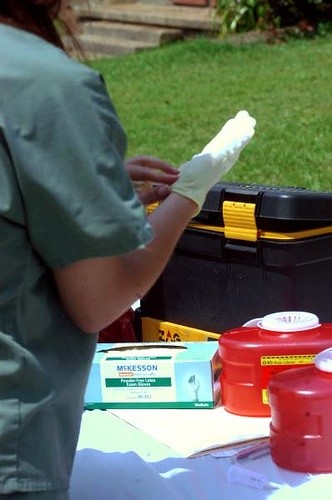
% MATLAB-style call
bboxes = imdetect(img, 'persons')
[0,0,256,500]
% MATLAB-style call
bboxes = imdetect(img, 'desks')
[67,402,332,500]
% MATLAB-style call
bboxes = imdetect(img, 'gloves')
[161,110,257,219]
